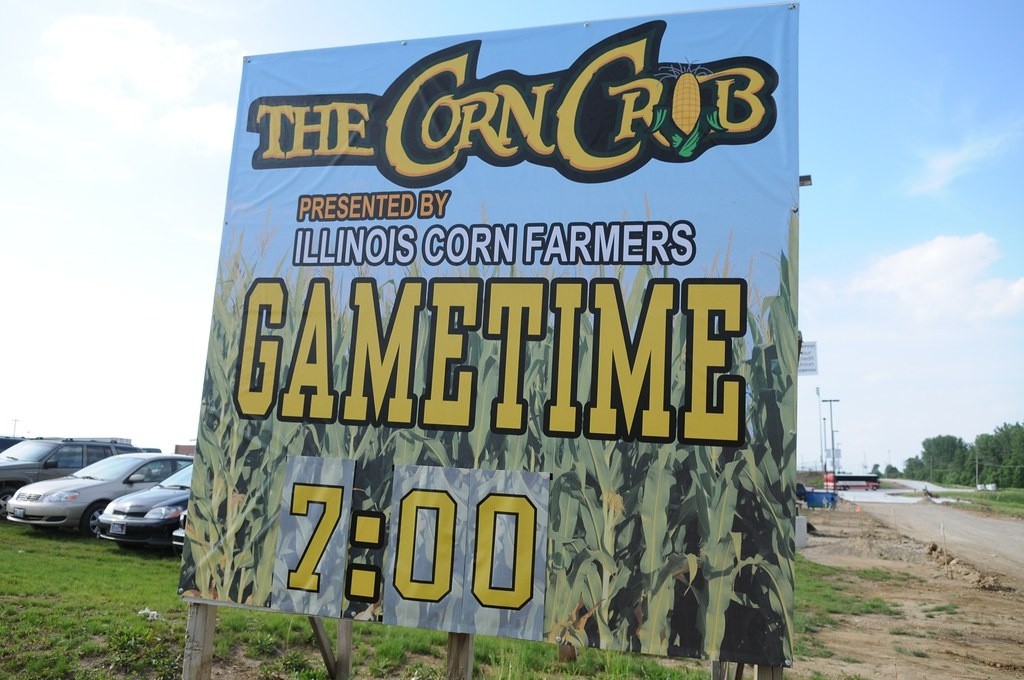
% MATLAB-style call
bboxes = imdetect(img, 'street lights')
[822,400,839,492]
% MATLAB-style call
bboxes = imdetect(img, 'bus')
[823,474,880,491]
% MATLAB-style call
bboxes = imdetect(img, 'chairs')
[142,467,151,482]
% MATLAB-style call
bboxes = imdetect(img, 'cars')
[0,437,153,518]
[7,452,195,537]
[97,463,194,548]
[172,510,187,556]
